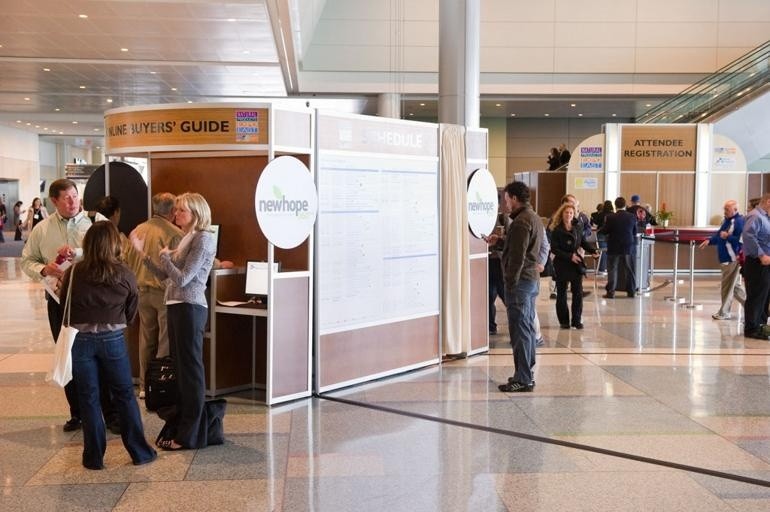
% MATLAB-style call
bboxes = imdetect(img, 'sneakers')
[712,313,730,319]
[498,377,534,392]
[63,417,81,432]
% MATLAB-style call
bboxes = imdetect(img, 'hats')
[631,195,639,202]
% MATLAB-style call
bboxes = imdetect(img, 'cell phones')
[481,234,491,242]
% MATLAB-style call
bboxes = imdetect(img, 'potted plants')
[656,202,673,227]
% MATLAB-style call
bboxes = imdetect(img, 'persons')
[699,193,769,340]
[1,198,50,243]
[547,147,559,170]
[19,180,219,469]
[557,143,570,167]
[481,181,658,393]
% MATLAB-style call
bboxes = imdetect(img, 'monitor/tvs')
[244,259,282,304]
[211,224,221,258]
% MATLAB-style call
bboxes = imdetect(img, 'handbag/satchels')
[204,399,227,445]
[145,355,178,410]
[45,325,80,389]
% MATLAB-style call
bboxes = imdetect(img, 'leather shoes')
[159,440,183,450]
